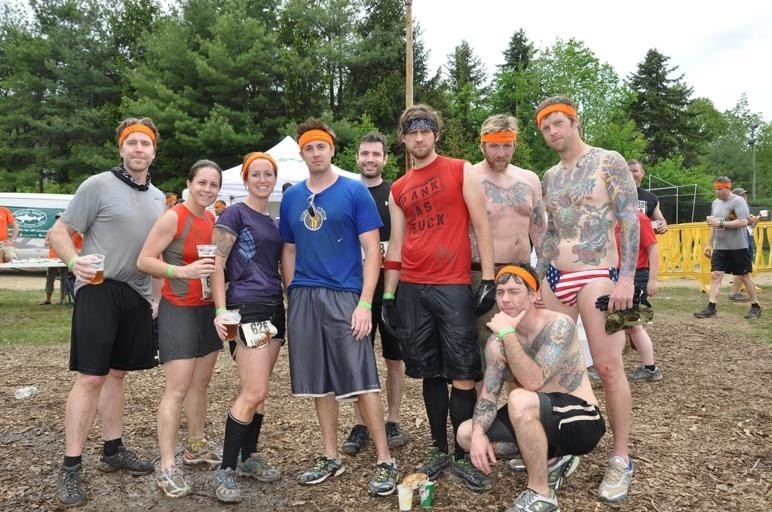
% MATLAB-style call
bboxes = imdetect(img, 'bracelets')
[11,240,16,242]
[719,220,724,229]
[499,327,517,341]
[358,301,372,311]
[383,293,394,299]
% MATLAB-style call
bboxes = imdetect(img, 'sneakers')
[626,367,665,382]
[296,455,347,487]
[727,292,748,301]
[542,454,581,492]
[95,447,153,477]
[505,487,562,512]
[693,308,718,318]
[180,440,225,466]
[746,306,762,319]
[340,424,372,456]
[450,452,495,493]
[234,451,283,484]
[55,463,88,507]
[598,451,634,503]
[153,460,193,499]
[366,456,401,496]
[211,463,244,504]
[383,421,408,450]
[417,445,451,480]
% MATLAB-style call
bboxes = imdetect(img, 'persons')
[535,97,667,499]
[44,118,166,507]
[729,187,768,302]
[464,113,549,392]
[383,104,496,490]
[693,176,762,319]
[457,264,606,512]
[137,159,224,499]
[0,206,19,263]
[343,133,405,456]
[211,152,284,502]
[280,120,399,494]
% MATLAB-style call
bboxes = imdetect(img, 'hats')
[733,186,751,195]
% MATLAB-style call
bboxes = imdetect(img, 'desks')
[1,259,68,305]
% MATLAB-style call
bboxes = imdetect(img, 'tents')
[183,136,359,215]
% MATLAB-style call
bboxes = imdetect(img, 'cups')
[706,216,713,226]
[397,485,413,510]
[420,480,434,507]
[219,310,241,341]
[652,220,663,234]
[196,244,217,274]
[83,254,105,285]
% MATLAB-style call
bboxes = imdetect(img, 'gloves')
[472,278,498,316]
[379,297,400,331]
[593,291,645,311]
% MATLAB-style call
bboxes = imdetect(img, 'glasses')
[306,192,318,217]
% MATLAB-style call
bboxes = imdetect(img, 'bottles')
[14,386,38,400]
